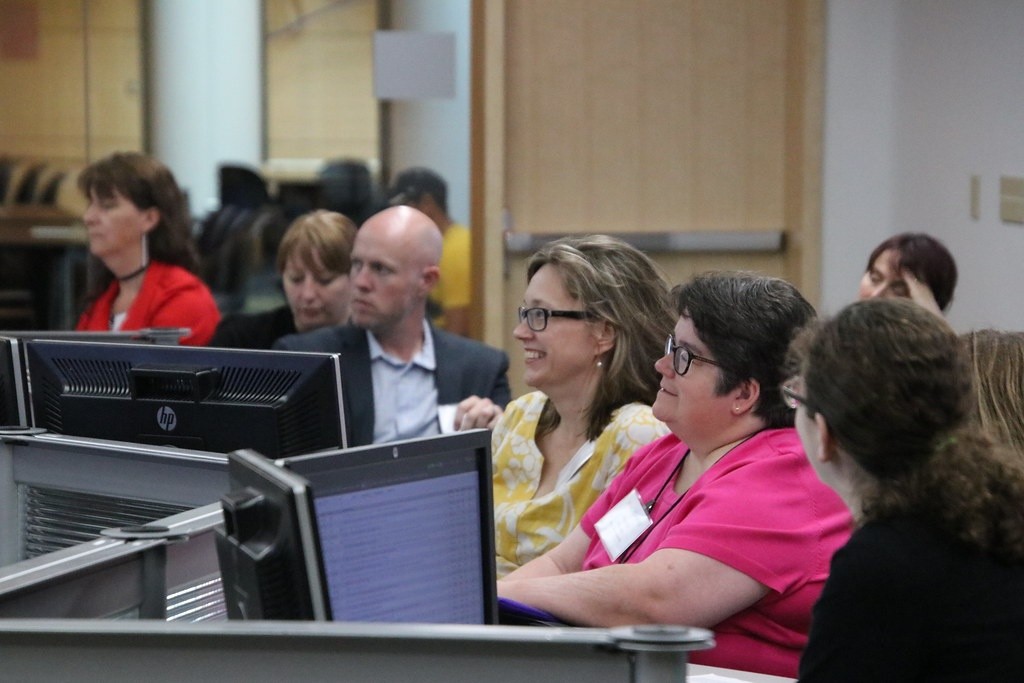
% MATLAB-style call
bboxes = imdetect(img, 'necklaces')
[116,259,152,282]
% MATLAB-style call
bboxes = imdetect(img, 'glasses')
[780,377,831,434]
[518,306,594,332]
[664,333,747,376]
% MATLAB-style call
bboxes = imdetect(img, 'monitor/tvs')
[0,335,34,430]
[213,448,332,622]
[25,338,351,459]
[273,426,498,625]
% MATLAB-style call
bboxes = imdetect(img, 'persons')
[63,147,1024,683]
